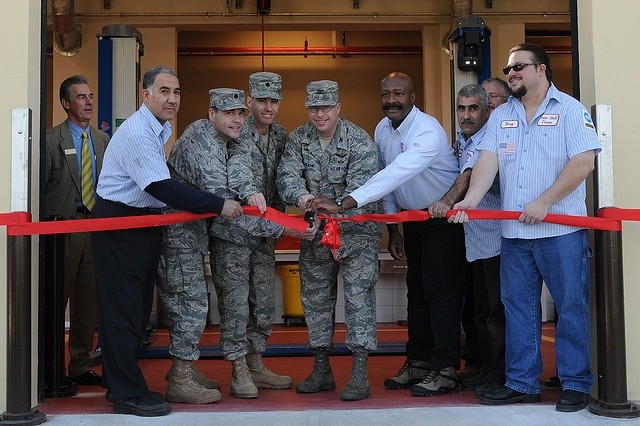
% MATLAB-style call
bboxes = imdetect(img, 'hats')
[249,72,283,99]
[305,80,339,107]
[209,88,249,111]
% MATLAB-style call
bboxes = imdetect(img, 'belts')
[97,196,164,214]
[77,206,92,214]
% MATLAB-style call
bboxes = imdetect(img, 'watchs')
[334,197,345,214]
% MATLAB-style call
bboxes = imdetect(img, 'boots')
[166,358,221,404]
[230,356,258,398]
[342,354,370,400]
[296,352,336,392]
[246,353,292,389]
[193,361,220,389]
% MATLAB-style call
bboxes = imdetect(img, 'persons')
[207,69,295,400]
[479,76,511,118]
[155,86,318,406]
[44,73,111,399]
[427,83,505,396]
[276,77,381,403]
[304,69,464,398]
[89,64,245,418]
[446,43,604,416]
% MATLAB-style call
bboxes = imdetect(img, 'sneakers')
[384,359,431,389]
[411,366,462,396]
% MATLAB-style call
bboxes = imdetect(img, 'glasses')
[306,103,339,113]
[502,63,541,75]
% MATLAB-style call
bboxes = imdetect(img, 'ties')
[82,132,95,210]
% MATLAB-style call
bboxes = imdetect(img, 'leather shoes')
[113,394,172,416]
[462,371,490,391]
[475,379,506,398]
[461,365,476,378]
[79,370,103,384]
[106,390,164,402]
[481,386,541,405]
[556,389,591,412]
